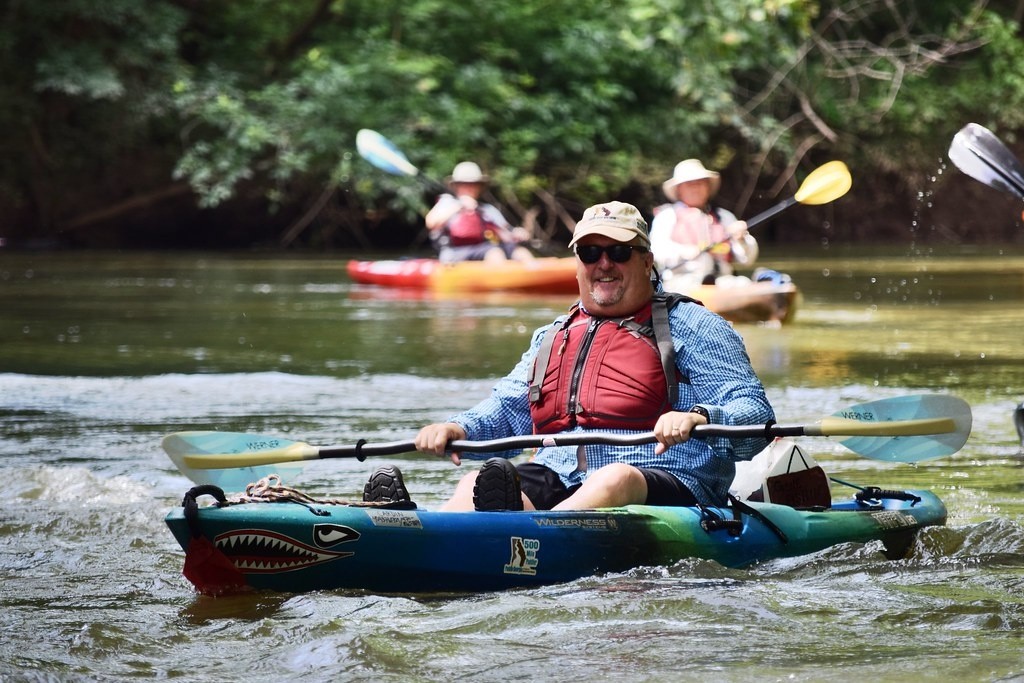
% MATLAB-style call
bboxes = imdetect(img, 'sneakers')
[363,466,410,504]
[473,456,523,511]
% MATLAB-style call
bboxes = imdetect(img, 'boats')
[665,280,798,324]
[348,255,579,294]
[164,483,947,596]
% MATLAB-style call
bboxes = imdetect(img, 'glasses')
[572,244,649,264]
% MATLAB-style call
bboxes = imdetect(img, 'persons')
[363,200,777,511]
[426,161,535,260]
[649,158,759,285]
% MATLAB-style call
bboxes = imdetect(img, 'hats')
[662,158,720,201]
[445,162,490,188]
[567,201,651,250]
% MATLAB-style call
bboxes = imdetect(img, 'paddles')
[948,121,1024,199]
[658,157,853,280]
[162,393,972,495]
[356,129,553,253]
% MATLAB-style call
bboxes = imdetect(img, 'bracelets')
[690,406,710,424]
